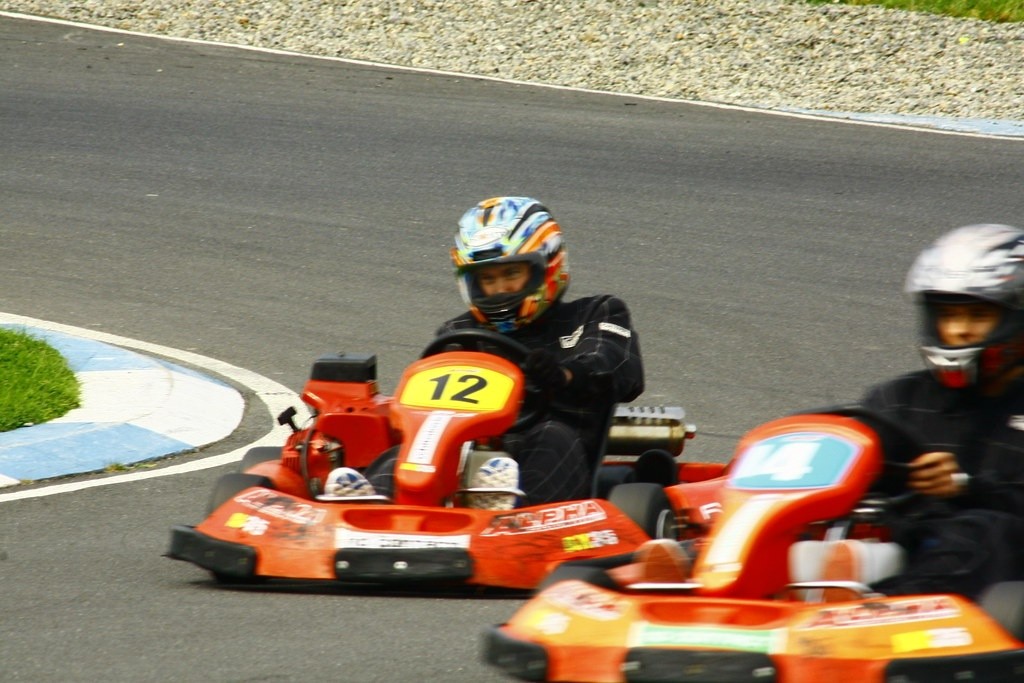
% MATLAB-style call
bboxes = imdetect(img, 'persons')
[324,195,645,511]
[639,224,1024,600]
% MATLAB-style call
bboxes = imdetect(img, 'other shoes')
[820,539,859,602]
[465,456,523,510]
[641,538,687,583]
[325,468,375,497]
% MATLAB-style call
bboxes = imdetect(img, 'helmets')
[903,223,1024,386]
[450,197,571,333]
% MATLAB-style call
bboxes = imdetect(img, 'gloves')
[522,348,567,390]
